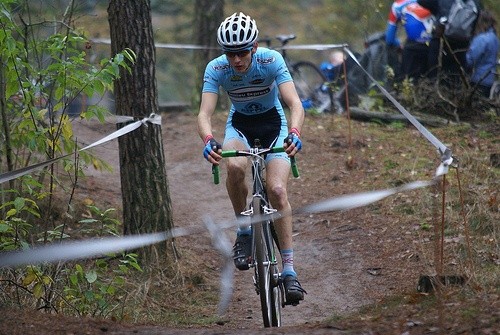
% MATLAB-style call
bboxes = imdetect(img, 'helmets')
[216,11,259,51]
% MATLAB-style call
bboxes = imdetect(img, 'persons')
[196,11,308,301]
[313,51,347,115]
[384,0,500,98]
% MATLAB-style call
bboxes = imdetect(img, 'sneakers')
[231,227,252,270]
[284,275,307,301]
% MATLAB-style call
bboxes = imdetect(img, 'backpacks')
[445,0,478,38]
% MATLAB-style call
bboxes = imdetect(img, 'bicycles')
[257,34,334,116]
[211,147,299,329]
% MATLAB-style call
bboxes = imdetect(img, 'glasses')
[223,46,253,58]
